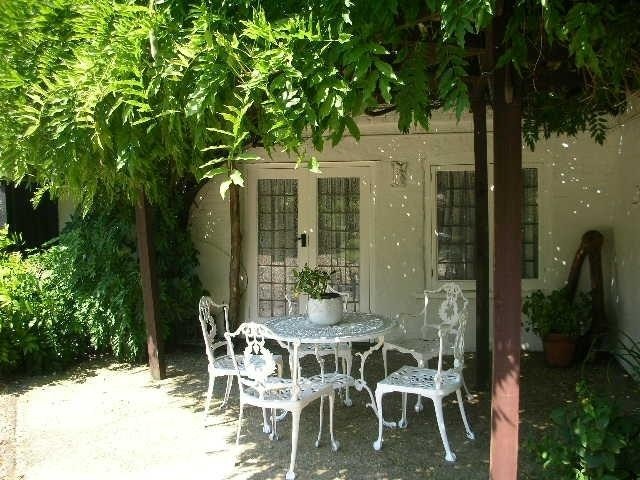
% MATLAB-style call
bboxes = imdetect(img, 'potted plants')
[290,264,347,326]
[520,286,596,367]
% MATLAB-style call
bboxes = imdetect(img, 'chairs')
[369,309,477,463]
[196,297,287,438]
[380,282,477,415]
[284,283,357,406]
[224,320,340,479]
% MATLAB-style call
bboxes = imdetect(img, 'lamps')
[390,159,408,187]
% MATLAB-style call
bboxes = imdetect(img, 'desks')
[250,310,403,451]
